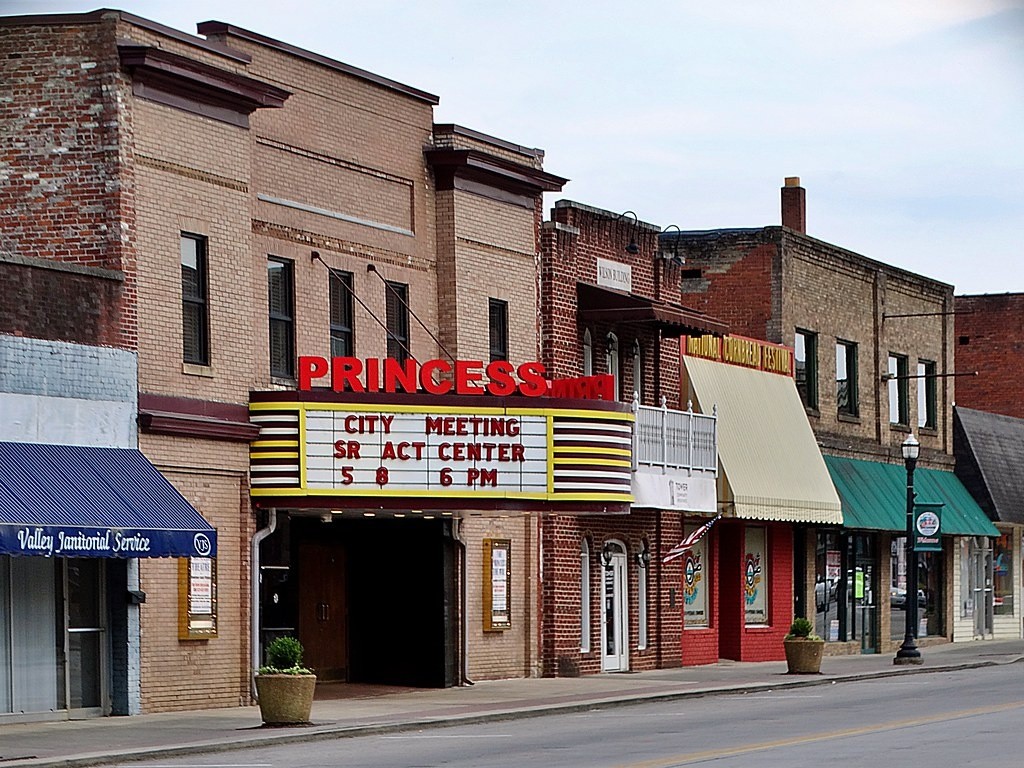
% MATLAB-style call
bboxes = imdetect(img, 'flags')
[664,511,722,562]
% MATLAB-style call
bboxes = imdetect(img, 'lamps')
[638,225,685,267]
[592,211,639,255]
[596,543,615,569]
[634,545,652,569]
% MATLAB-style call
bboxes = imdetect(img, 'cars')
[814,573,928,613]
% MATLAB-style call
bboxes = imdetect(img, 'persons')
[0,441,217,558]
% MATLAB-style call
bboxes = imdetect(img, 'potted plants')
[783,618,824,674]
[253,638,317,724]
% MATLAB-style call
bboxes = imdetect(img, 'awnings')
[953,407,1024,526]
[822,454,1001,540]
[681,357,843,523]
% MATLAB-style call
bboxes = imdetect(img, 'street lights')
[889,432,926,664]
